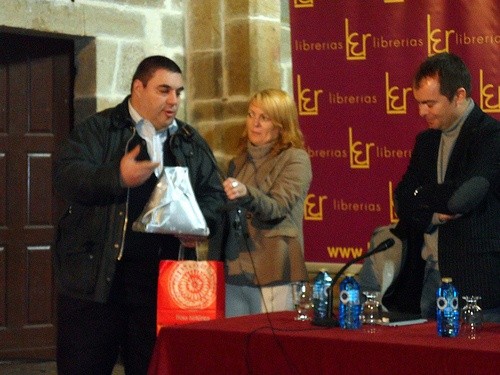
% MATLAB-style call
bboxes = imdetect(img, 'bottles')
[312,269,334,326]
[339,273,361,328]
[435,278,459,336]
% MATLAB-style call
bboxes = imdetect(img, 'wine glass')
[292,281,312,322]
[460,295,484,339]
[358,291,384,332]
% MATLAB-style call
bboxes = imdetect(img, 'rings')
[232,181,238,187]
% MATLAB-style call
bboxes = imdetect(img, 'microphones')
[320,237,396,329]
[227,161,248,238]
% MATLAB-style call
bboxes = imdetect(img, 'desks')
[143,308,500,375]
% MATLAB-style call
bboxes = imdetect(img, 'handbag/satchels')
[225,198,248,260]
[157,235,226,337]
[360,222,404,290]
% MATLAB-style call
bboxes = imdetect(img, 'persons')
[220,88,313,319]
[51,56,226,375]
[382,52,500,313]
